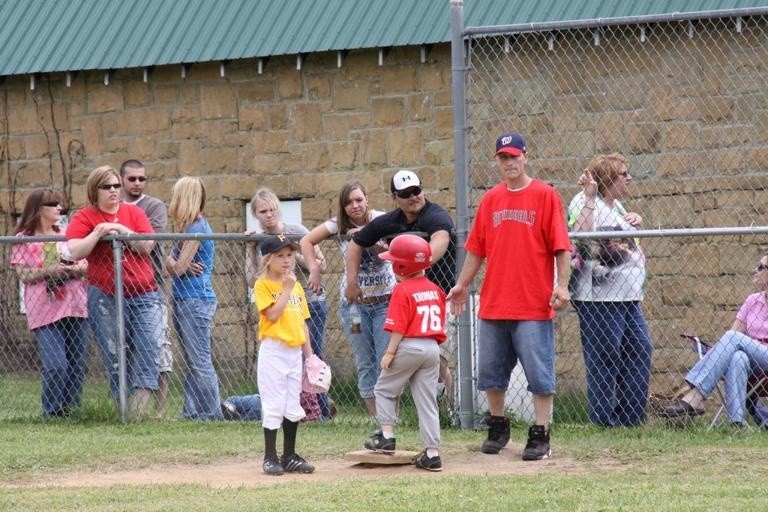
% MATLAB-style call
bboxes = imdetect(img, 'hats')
[391,171,421,193]
[261,235,291,255]
[494,134,526,159]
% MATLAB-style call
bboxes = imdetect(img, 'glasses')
[98,184,121,189]
[43,201,60,206]
[395,189,420,197]
[128,176,145,181]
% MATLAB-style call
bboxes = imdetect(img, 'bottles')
[54,249,76,285]
[349,300,362,335]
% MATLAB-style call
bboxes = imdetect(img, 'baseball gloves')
[302,353,332,393]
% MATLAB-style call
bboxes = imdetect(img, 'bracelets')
[584,205,596,210]
[385,351,394,356]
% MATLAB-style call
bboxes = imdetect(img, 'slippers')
[661,400,704,417]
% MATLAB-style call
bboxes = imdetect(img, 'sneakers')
[263,453,314,475]
[481,415,510,454]
[523,424,551,460]
[415,453,441,470]
[365,431,395,452]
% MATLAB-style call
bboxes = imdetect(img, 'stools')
[681,329,767,432]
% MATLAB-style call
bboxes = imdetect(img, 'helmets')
[378,235,433,276]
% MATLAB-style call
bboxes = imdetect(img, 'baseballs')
[551,297,561,305]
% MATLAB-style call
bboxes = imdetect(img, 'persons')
[658,254,768,436]
[66,166,160,414]
[252,234,334,475]
[565,151,655,426]
[166,175,224,422]
[9,189,89,416]
[299,180,404,426]
[364,233,446,471]
[117,159,173,420]
[445,132,573,462]
[245,186,330,419]
[344,170,457,410]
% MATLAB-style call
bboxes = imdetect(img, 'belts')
[362,294,392,304]
